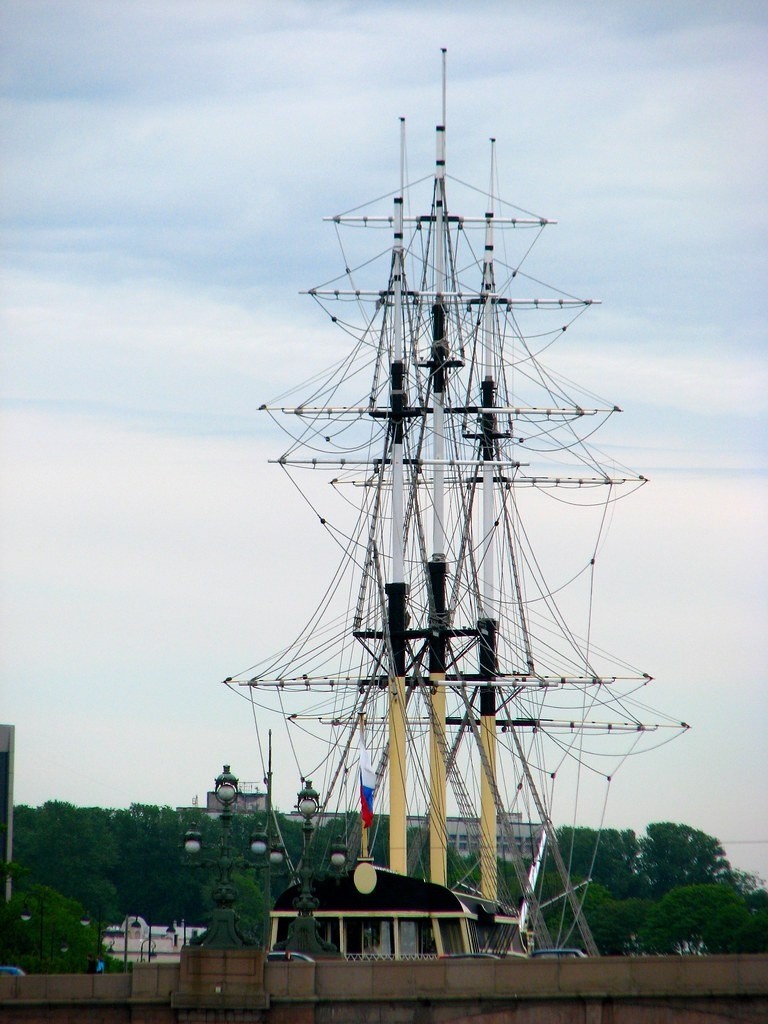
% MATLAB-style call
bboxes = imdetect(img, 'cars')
[269,952,316,962]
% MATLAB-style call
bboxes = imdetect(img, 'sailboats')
[222,48,679,962]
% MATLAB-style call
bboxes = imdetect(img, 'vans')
[439,953,502,962]
[529,950,589,961]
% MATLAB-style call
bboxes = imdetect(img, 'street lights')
[131,903,152,963]
[270,781,348,953]
[178,766,269,947]
[79,898,101,956]
[138,938,157,962]
[21,892,43,958]
[166,914,187,945]
